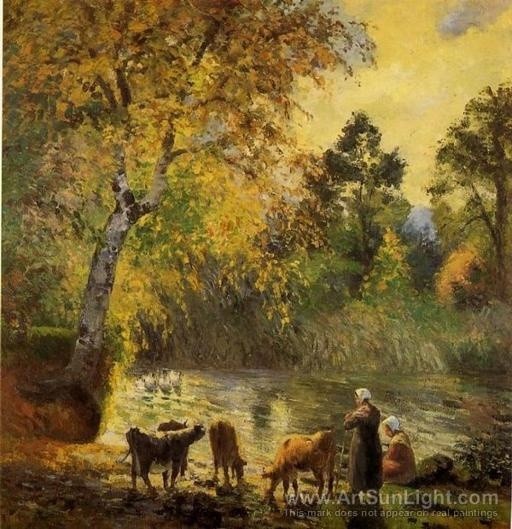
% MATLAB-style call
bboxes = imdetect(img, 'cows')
[119,417,206,490]
[259,429,337,502]
[207,419,248,484]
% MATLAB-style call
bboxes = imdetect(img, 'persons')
[342,388,384,506]
[383,415,416,485]
